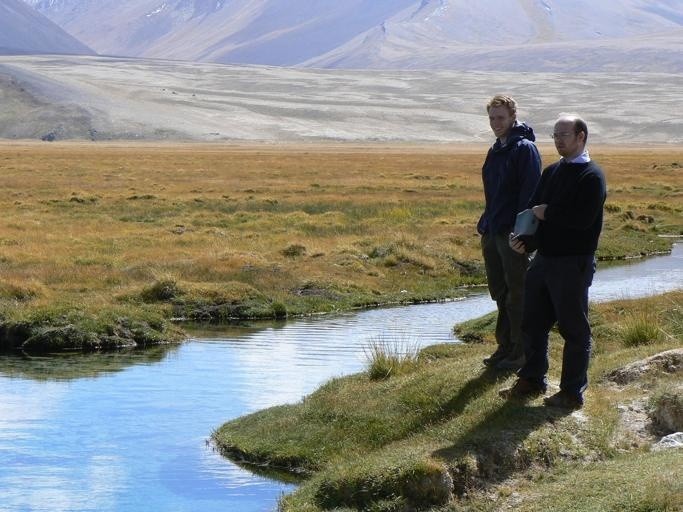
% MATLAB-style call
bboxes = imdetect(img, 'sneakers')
[499,378,546,396]
[484,350,520,370]
[544,391,583,407]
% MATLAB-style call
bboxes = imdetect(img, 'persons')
[475,95,543,370]
[495,114,608,409]
